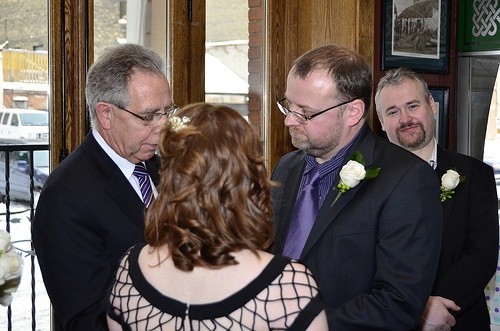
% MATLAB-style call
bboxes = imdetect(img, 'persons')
[374,69,499,331]
[32,46,180,331]
[263,46,438,331]
[106,103,330,331]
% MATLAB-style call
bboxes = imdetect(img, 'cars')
[0,138,50,208]
[0,105,48,141]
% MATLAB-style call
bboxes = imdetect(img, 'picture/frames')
[379,0,451,75]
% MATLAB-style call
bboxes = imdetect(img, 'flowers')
[0,229,22,307]
[330,151,382,208]
[440,166,466,202]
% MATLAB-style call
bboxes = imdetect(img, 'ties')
[282,166,321,261]
[134,162,156,212]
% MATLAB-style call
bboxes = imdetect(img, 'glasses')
[277,98,360,124]
[105,100,180,126]
